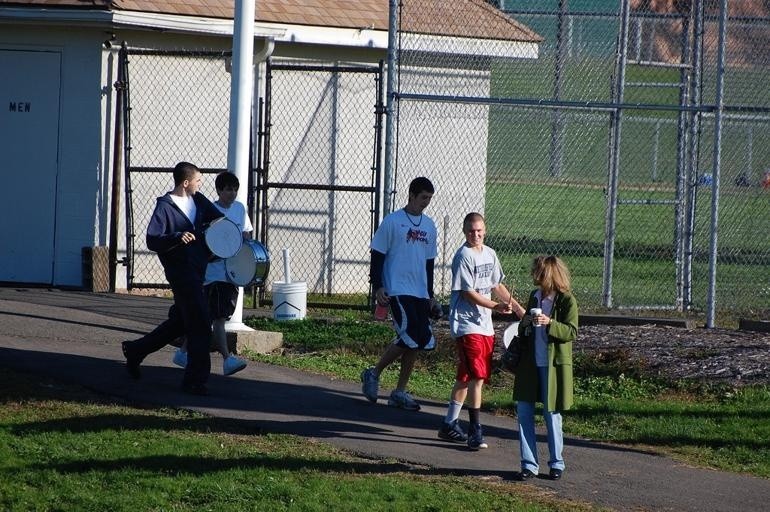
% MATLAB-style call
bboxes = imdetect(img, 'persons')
[513,254,577,483]
[172,172,254,377]
[438,212,526,451]
[121,162,225,397]
[359,176,438,410]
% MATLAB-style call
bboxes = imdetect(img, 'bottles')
[374,291,391,322]
[427,303,441,326]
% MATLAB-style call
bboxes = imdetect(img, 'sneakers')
[120,339,147,382]
[386,389,421,411]
[222,355,246,376]
[359,367,380,404]
[173,348,189,368]
[467,423,488,450]
[180,380,208,397]
[436,415,469,443]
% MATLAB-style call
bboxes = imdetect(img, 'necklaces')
[404,206,423,227]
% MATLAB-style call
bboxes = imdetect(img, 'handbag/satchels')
[501,335,525,376]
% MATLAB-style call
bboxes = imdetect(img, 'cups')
[530,307,543,327]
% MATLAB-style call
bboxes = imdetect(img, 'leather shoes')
[549,466,562,480]
[517,468,535,479]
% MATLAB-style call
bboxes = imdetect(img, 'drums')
[502,321,522,373]
[225,239,269,287]
[199,218,242,264]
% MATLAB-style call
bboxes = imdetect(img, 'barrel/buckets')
[272,281,308,321]
